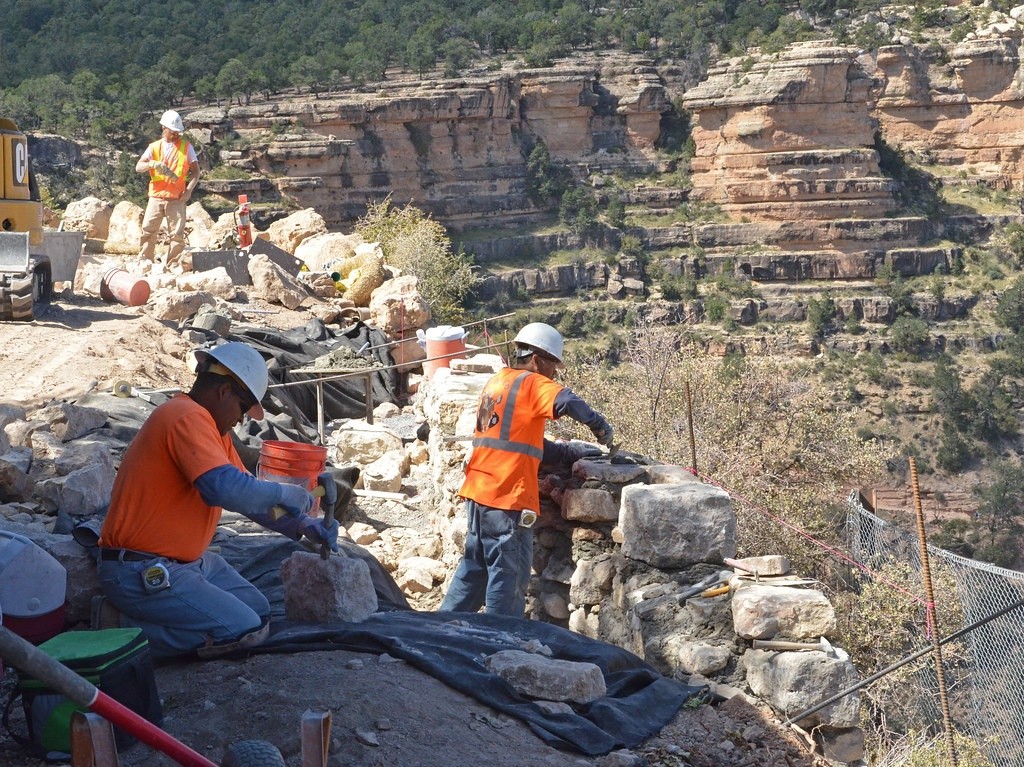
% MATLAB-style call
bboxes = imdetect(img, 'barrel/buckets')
[256,439,327,514]
[101,268,150,306]
[425,325,470,380]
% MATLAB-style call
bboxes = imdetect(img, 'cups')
[165,168,178,182]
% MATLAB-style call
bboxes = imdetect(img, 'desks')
[291,365,373,426]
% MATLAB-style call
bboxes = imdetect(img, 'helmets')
[195,342,269,420]
[511,322,565,369]
[159,110,185,132]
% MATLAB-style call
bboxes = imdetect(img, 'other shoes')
[90,595,121,632]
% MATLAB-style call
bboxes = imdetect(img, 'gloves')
[298,513,340,552]
[273,482,314,519]
[148,160,167,174]
[590,419,614,448]
[561,441,603,464]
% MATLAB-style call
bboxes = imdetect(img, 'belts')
[100,548,156,562]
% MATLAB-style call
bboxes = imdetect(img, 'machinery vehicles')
[0,117,87,322]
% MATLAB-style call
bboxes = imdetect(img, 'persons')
[135,109,200,266]
[90,341,340,660]
[438,322,614,621]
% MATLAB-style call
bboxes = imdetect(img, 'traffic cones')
[238,193,253,249]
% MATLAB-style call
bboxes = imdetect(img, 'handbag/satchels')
[2,624,164,765]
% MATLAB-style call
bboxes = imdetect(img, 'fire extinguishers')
[233,201,252,249]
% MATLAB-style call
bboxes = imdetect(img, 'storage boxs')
[17,627,165,755]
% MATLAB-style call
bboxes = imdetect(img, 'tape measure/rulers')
[140,563,171,591]
[516,509,537,528]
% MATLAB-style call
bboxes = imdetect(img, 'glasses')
[229,389,251,415]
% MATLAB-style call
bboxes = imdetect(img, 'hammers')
[722,557,760,584]
[751,635,835,657]
[267,472,338,522]
[596,428,623,460]
